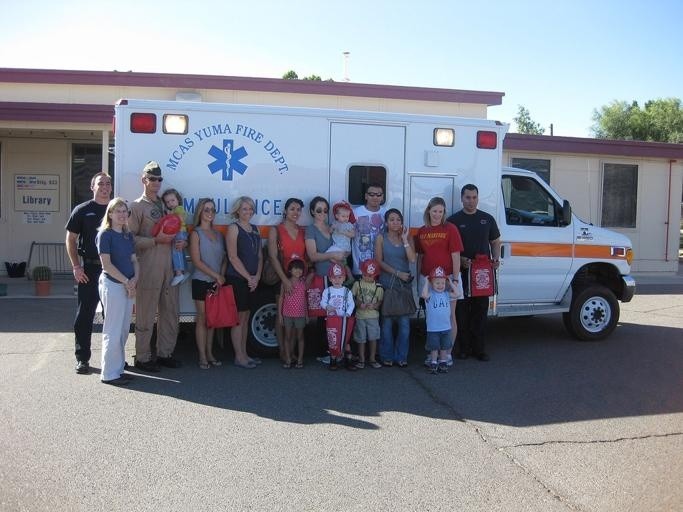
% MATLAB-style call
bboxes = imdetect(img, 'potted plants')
[32,265,52,297]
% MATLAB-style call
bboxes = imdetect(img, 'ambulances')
[111,93,638,363]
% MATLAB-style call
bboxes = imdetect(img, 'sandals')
[206,357,224,368]
[197,357,210,370]
[279,353,304,370]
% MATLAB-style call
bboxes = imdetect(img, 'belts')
[85,257,102,265]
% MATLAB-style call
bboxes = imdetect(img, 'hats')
[359,259,381,275]
[327,263,348,277]
[143,160,161,176]
[427,265,449,279]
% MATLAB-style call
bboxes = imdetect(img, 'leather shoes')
[135,359,161,373]
[233,356,263,369]
[156,353,178,370]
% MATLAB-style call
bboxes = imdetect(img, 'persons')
[65,161,500,385]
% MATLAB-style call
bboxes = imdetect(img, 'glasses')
[145,177,163,182]
[367,192,384,196]
[203,208,217,213]
[315,208,328,214]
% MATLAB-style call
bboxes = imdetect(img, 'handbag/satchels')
[260,224,285,286]
[324,307,356,358]
[381,270,417,316]
[467,253,499,299]
[305,272,328,318]
[204,282,240,329]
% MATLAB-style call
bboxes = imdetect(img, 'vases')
[4,261,26,278]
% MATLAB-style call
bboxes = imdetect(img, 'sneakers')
[179,272,191,286]
[424,346,490,375]
[327,359,408,372]
[171,273,184,287]
[75,358,90,374]
[101,372,136,386]
